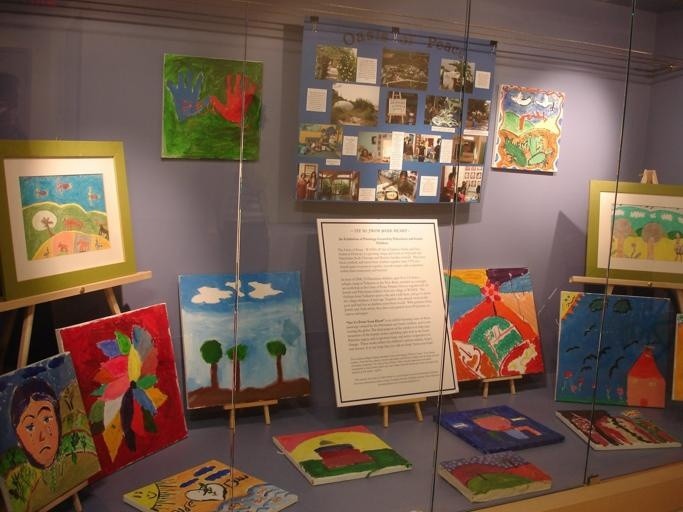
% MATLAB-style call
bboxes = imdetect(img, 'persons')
[3,378,61,512]
[297,97,491,203]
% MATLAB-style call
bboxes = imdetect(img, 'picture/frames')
[585,180,683,285]
[0,138,136,302]
